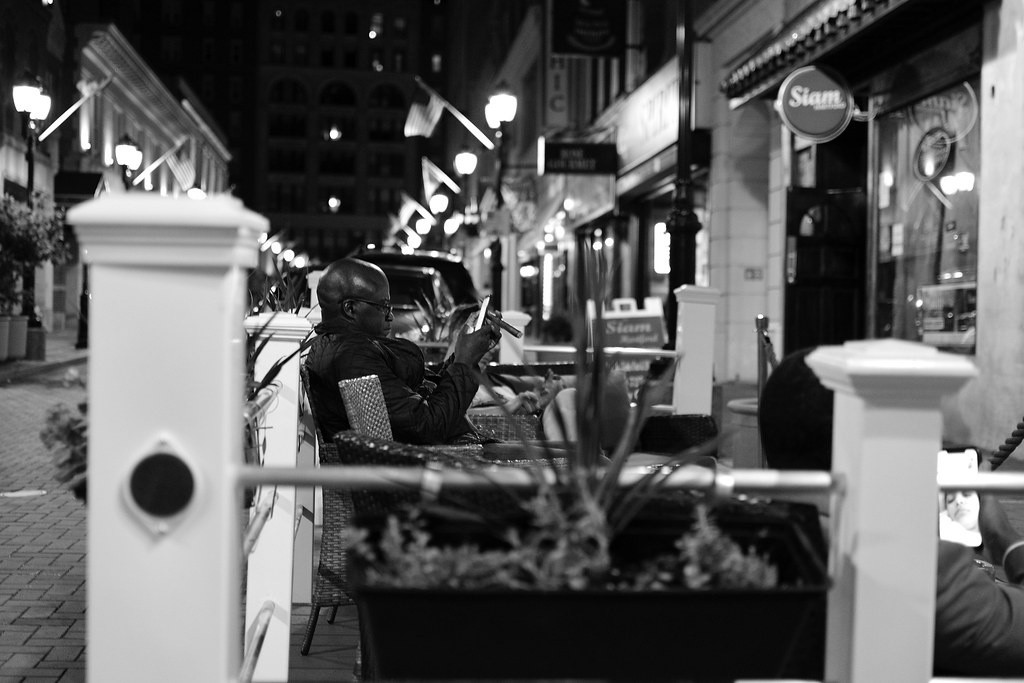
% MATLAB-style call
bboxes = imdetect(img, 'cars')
[354,246,484,340]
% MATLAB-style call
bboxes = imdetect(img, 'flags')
[382,156,446,251]
[404,76,447,137]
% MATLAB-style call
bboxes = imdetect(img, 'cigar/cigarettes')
[485,308,523,339]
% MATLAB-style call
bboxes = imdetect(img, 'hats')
[758,350,834,471]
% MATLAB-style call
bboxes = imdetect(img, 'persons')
[945,489,979,531]
[756,347,1024,683]
[304,258,613,467]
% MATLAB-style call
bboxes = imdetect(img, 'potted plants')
[343,245,831,683]
[0,191,70,362]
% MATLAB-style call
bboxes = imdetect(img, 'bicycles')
[400,285,526,356]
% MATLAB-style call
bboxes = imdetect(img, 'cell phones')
[475,294,492,332]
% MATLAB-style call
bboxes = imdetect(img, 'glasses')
[351,298,391,321]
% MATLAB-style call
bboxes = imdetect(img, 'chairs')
[298,365,565,654]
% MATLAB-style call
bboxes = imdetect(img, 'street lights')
[115,133,144,192]
[479,79,518,321]
[11,68,53,329]
